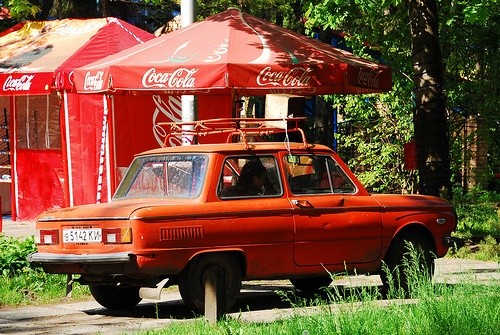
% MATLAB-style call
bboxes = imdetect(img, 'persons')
[223,160,270,197]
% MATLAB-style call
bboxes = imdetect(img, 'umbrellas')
[74,7,393,143]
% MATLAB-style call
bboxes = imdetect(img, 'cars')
[27,115,459,316]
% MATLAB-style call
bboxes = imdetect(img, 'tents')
[0,17,237,223]
